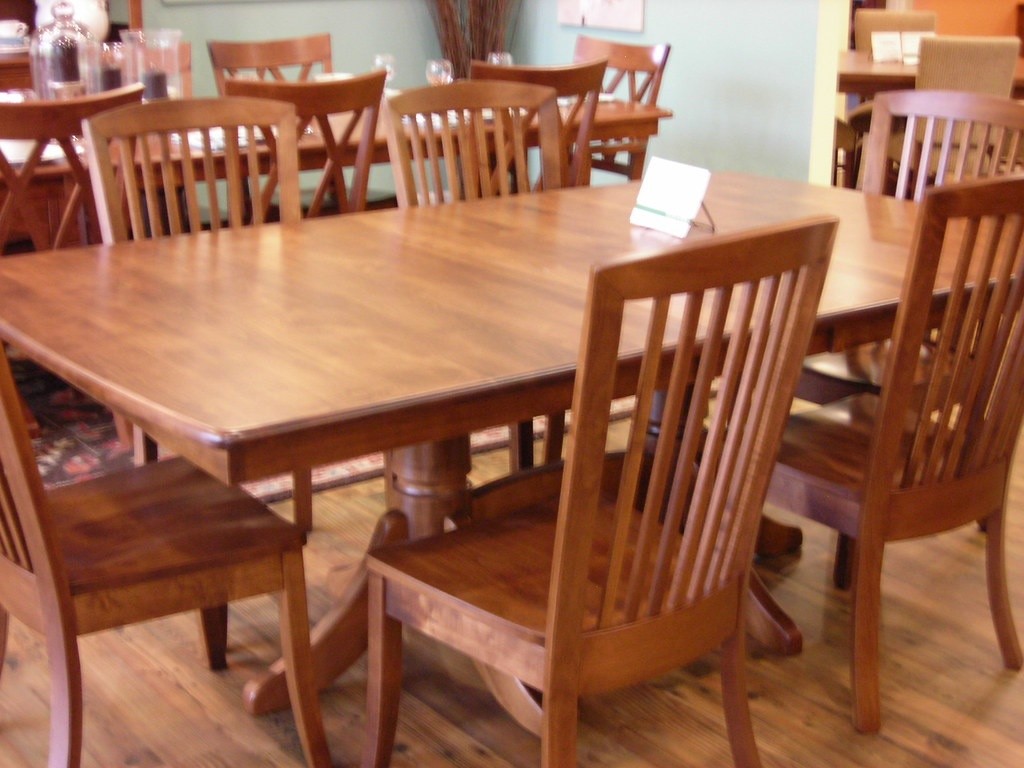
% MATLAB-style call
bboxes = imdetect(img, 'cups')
[29,1,183,144]
[425,59,453,86]
[370,51,393,94]
[487,52,514,68]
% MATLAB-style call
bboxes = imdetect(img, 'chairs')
[0,10,1024,767]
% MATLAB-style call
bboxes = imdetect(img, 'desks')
[0,51,166,96]
[0,172,1023,735]
[0,93,675,256]
[836,50,1024,99]
